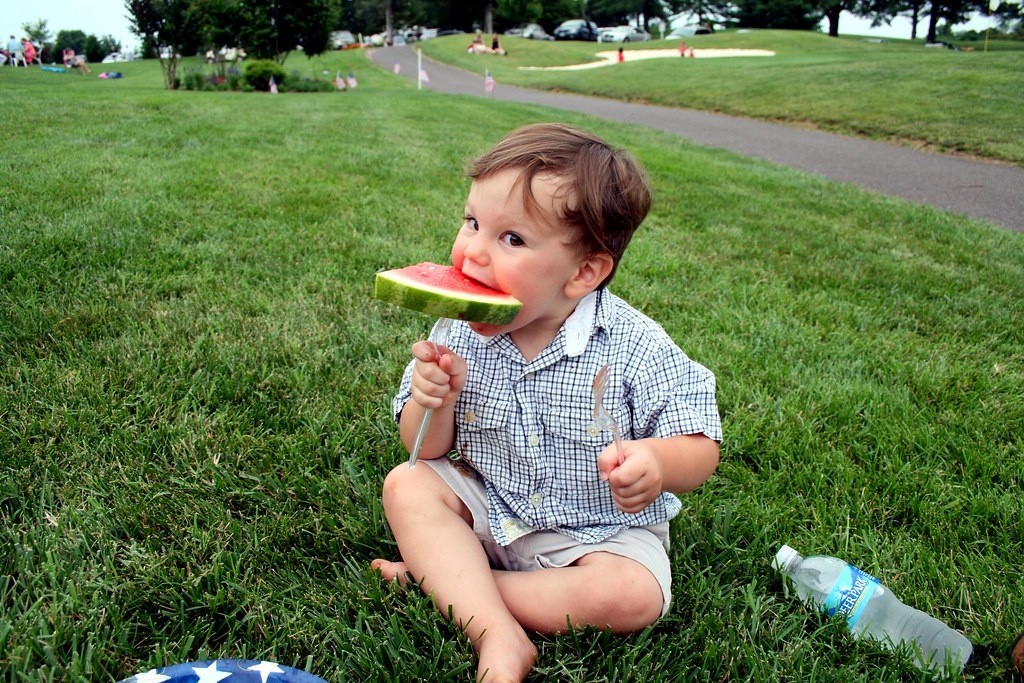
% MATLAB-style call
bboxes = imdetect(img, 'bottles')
[771,544,973,681]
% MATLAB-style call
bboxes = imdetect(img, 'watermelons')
[375,262,524,325]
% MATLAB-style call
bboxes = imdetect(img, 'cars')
[925,41,957,51]
[438,30,465,36]
[205,46,247,63]
[363,27,438,47]
[134,48,166,59]
[0,53,11,67]
[0,49,11,65]
[553,19,598,42]
[101,52,134,63]
[665,25,713,40]
[601,25,652,42]
[504,23,550,40]
[76,54,87,63]
[598,27,616,43]
[160,45,182,58]
[296,31,355,50]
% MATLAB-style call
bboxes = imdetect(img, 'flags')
[488,72,494,92]
[394,62,400,73]
[347,74,357,88]
[269,78,277,93]
[337,73,345,88]
[421,66,429,81]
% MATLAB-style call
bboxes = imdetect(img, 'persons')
[111,46,118,62]
[125,45,133,61]
[372,122,723,683]
[618,47,624,61]
[7,35,36,66]
[206,45,244,63]
[467,33,507,55]
[99,72,123,79]
[678,41,693,57]
[64,48,92,75]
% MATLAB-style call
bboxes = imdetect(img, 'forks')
[406,316,454,470]
[592,361,624,467]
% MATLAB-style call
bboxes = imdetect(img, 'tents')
[103,53,127,62]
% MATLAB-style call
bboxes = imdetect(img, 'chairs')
[9,46,82,73]
